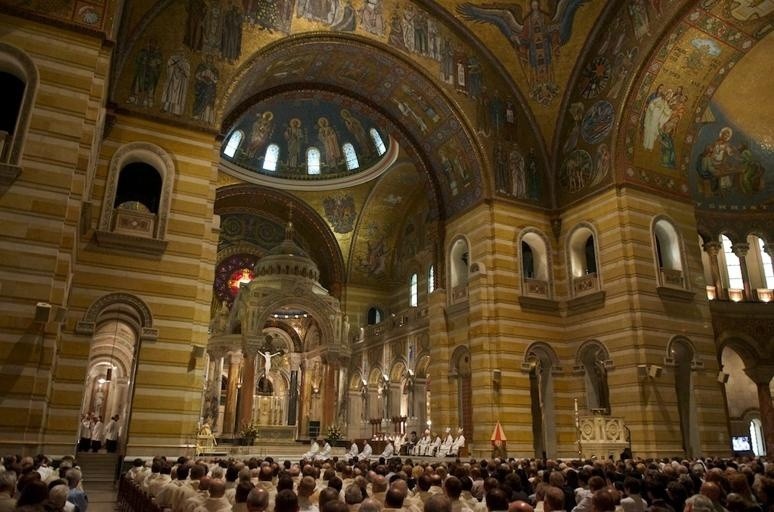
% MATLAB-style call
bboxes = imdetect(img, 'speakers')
[491,369,501,383]
[636,365,663,379]
[717,371,730,383]
[33,302,68,324]
[190,343,205,360]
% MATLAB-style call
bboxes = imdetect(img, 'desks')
[371,441,395,455]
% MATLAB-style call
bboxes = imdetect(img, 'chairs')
[117,473,172,512]
[431,440,468,458]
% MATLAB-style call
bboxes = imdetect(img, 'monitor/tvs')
[731,436,751,451]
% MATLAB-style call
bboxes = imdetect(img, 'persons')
[371,426,465,448]
[342,438,359,460]
[255,349,280,377]
[0,452,87,511]
[355,439,373,460]
[312,439,332,460]
[303,437,319,461]
[127,450,773,511]
[76,412,120,453]
